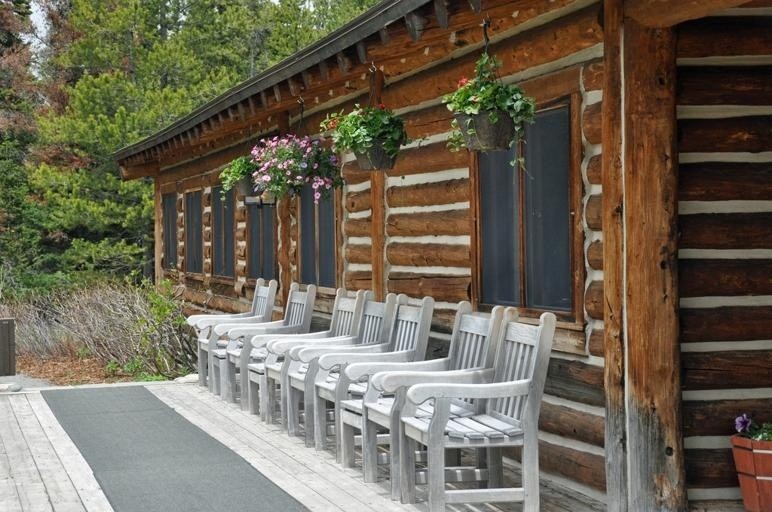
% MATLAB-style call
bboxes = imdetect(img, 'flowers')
[442,51,535,184]
[320,103,412,170]
[736,413,772,441]
[250,134,344,206]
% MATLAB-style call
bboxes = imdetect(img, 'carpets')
[41,386,312,512]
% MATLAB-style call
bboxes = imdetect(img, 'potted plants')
[218,156,262,210]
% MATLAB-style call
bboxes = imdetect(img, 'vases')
[353,139,394,170]
[454,110,514,151]
[731,435,772,512]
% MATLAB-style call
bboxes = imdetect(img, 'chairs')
[187,278,557,512]
